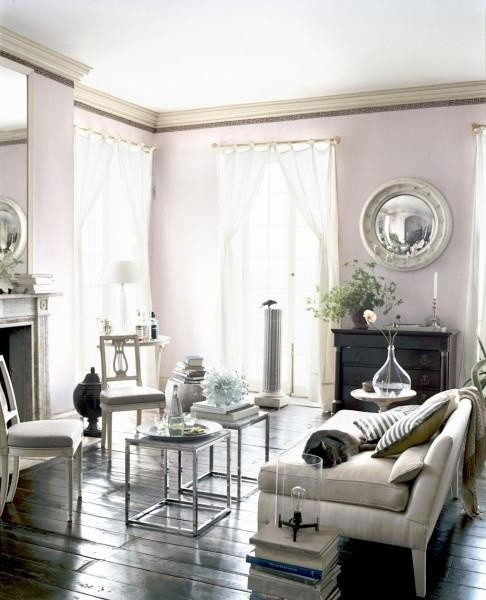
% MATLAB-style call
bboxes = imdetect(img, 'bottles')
[135,311,144,341]
[168,384,184,437]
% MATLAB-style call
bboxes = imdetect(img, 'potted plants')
[302,258,405,329]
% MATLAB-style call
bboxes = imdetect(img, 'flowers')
[362,310,404,395]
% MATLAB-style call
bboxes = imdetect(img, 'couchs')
[256,397,471,597]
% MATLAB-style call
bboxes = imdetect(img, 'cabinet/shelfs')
[331,327,461,416]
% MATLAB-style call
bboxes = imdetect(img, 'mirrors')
[0,52,37,294]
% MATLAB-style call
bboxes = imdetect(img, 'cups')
[153,414,168,431]
[151,312,159,341]
[362,382,374,392]
[184,412,196,429]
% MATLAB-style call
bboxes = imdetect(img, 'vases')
[371,344,412,396]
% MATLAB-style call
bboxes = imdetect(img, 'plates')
[136,418,222,440]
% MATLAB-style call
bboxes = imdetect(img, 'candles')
[432,271,439,300]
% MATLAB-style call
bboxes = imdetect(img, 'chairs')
[99,335,167,462]
[0,355,87,523]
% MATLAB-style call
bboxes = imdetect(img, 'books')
[244,520,342,600]
[189,398,260,422]
[171,355,206,385]
[10,273,60,295]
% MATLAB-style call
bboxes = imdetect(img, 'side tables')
[96,339,160,378]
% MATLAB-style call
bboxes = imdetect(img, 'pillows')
[370,398,448,457]
[385,402,422,415]
[422,388,460,425]
[350,409,402,450]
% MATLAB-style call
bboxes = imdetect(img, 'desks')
[123,427,232,536]
[178,412,269,506]
